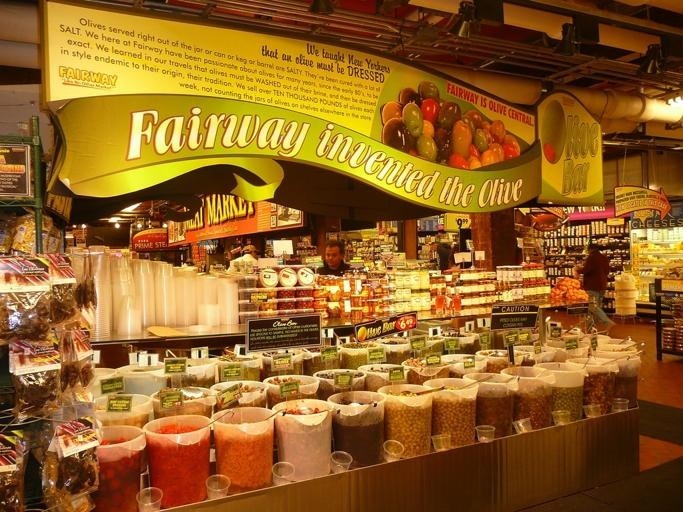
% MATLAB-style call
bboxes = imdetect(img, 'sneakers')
[606,322,617,332]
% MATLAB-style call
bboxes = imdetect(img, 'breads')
[613,240,683,315]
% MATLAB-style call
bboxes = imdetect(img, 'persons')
[227,244,260,275]
[576,243,617,335]
[318,239,351,276]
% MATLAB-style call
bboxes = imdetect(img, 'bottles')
[496,266,521,304]
[397,270,496,321]
[523,264,551,304]
[536,218,632,312]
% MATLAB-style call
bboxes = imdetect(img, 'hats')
[242,244,261,253]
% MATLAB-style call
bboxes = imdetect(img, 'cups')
[91,255,112,343]
[583,404,602,418]
[205,474,231,499]
[474,425,496,442]
[330,450,353,473]
[432,434,451,452]
[136,486,164,512]
[383,439,405,462]
[272,460,296,485]
[609,398,630,412]
[552,410,570,425]
[512,417,533,433]
[113,254,239,337]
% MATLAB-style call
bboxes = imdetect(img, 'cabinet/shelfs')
[546,205,628,312]
[656,279,683,360]
[629,217,682,318]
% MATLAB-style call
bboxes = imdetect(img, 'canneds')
[312,273,397,324]
